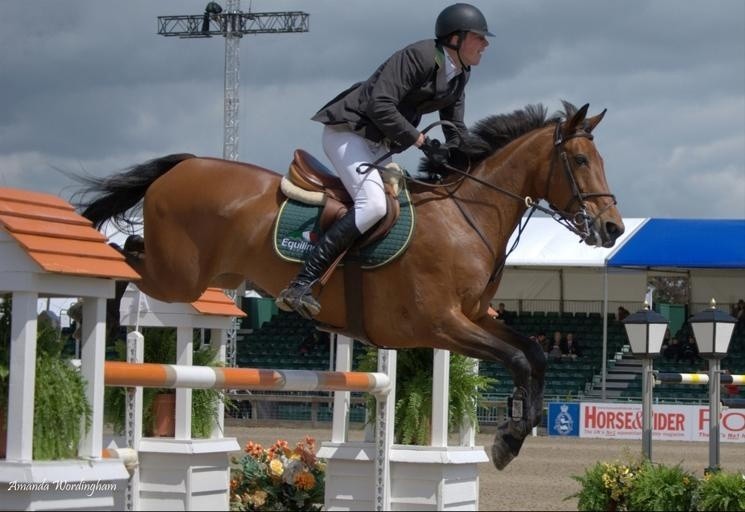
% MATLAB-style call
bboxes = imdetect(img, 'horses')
[48,98,626,471]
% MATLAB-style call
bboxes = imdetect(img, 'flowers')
[561,444,745,512]
[229,434,327,512]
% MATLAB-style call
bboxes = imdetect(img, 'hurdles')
[70,349,398,511]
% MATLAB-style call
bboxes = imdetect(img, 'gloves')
[419,134,451,165]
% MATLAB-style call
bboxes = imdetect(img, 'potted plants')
[102,326,240,438]
[0,317,94,462]
[357,347,502,445]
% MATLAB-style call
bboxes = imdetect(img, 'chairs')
[234,308,745,406]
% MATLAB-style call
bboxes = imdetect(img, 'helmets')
[435,4,497,45]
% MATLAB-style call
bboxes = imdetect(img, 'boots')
[275,206,363,317]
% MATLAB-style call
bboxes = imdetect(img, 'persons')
[275,3,496,321]
[619,307,629,320]
[662,313,699,364]
[494,302,511,326]
[549,329,563,362]
[562,333,579,360]
[537,333,551,360]
[294,313,336,355]
[732,299,745,336]
